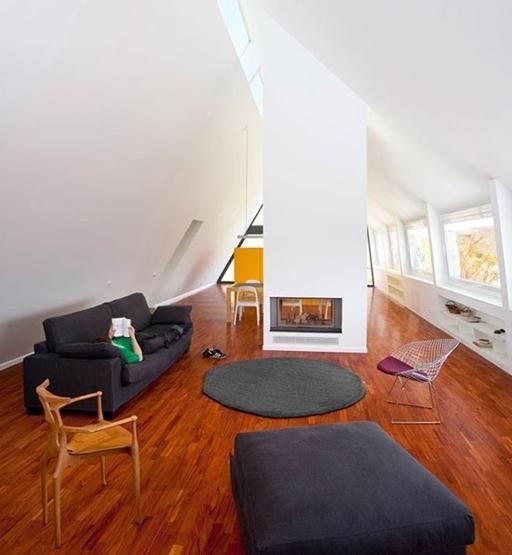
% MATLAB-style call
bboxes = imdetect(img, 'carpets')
[199,354,365,417]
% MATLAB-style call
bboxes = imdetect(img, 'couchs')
[24,291,193,416]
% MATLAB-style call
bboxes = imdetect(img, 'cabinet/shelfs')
[386,276,405,304]
[437,294,509,369]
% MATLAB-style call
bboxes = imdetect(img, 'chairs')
[36,377,141,549]
[234,279,261,327]
[374,337,463,426]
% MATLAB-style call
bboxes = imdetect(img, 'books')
[112,316,132,339]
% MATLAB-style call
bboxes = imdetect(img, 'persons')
[90,321,193,365]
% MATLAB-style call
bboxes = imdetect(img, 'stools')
[229,417,477,555]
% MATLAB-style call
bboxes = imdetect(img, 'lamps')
[236,126,263,241]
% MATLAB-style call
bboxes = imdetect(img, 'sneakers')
[202,348,227,359]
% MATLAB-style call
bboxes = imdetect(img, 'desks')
[227,282,262,322]
